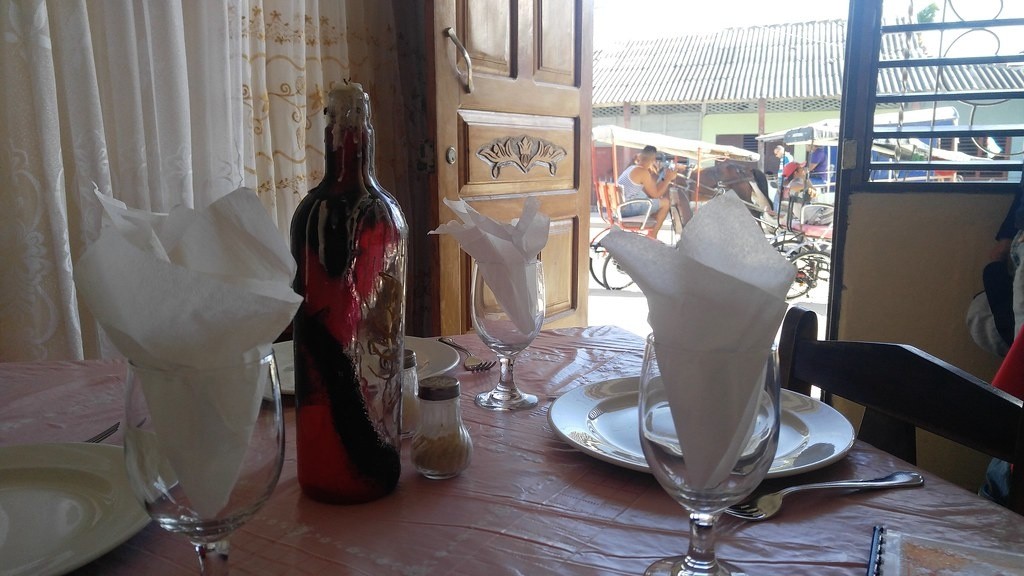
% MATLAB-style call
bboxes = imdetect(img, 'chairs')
[669,188,693,233]
[779,307,1024,516]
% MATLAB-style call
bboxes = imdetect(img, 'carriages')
[624,107,958,236]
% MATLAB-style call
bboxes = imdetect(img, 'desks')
[0,328,1024,576]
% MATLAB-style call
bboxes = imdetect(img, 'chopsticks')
[85,422,120,443]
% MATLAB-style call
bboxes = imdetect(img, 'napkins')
[600,189,798,489]
[427,194,551,333]
[73,182,304,519]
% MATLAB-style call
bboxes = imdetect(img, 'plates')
[547,376,857,480]
[263,335,460,394]
[0,442,152,576]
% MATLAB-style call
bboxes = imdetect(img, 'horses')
[625,153,773,235]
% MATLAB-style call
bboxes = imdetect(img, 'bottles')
[290,89,409,506]
[410,374,473,480]
[402,348,420,439]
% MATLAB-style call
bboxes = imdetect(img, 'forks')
[723,469,924,522]
[438,337,497,371]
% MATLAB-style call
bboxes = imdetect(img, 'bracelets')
[662,178,664,181]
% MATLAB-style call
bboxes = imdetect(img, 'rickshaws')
[589,139,995,299]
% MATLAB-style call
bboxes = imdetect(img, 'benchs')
[750,180,802,233]
[780,192,835,239]
[596,181,657,230]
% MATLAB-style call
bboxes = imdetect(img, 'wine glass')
[638,332,780,576]
[469,257,547,413]
[122,348,286,576]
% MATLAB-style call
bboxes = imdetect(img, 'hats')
[782,162,807,178]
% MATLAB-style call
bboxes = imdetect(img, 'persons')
[804,145,827,184]
[966,162,1024,517]
[618,145,678,239]
[773,145,793,219]
[783,162,817,197]
